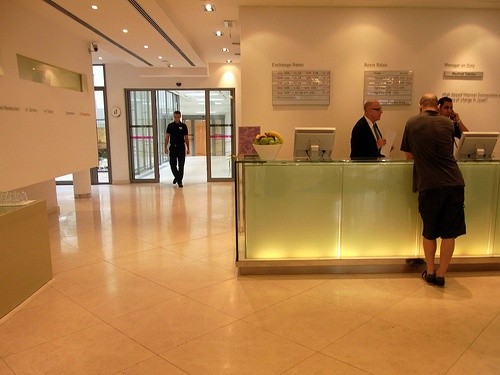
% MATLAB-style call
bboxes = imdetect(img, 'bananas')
[265,132,284,144]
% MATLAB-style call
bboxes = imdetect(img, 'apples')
[254,137,280,145]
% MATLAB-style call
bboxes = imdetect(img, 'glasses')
[368,108,381,113]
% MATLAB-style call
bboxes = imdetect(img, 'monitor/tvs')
[455,132,500,159]
[292,127,336,161]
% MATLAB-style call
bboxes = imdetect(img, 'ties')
[374,124,380,141]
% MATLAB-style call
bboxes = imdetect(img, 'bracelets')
[458,120,462,125]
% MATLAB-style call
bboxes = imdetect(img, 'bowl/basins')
[252,144,282,161]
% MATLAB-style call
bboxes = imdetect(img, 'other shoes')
[173,178,177,184]
[434,276,445,286]
[179,182,183,187]
[422,270,435,284]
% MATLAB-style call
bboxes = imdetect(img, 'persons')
[348,100,395,161]
[433,97,469,159]
[403,94,468,287]
[165,110,190,187]
